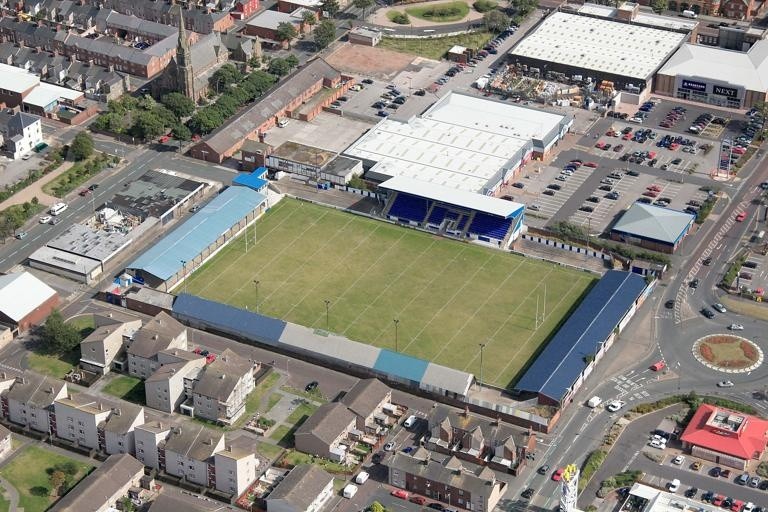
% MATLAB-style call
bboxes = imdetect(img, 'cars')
[17,14,31,22]
[205,354,216,364]
[200,349,209,356]
[135,43,149,50]
[192,347,201,354]
[342,357,768,511]
[14,131,201,240]
[329,9,768,330]
[305,381,318,392]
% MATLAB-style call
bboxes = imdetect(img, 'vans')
[279,120,289,128]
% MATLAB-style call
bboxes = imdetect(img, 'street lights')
[594,341,608,373]
[286,358,292,376]
[394,319,400,353]
[253,279,260,313]
[479,343,485,390]
[255,149,266,169]
[181,260,187,295]
[324,300,331,332]
[47,415,53,447]
